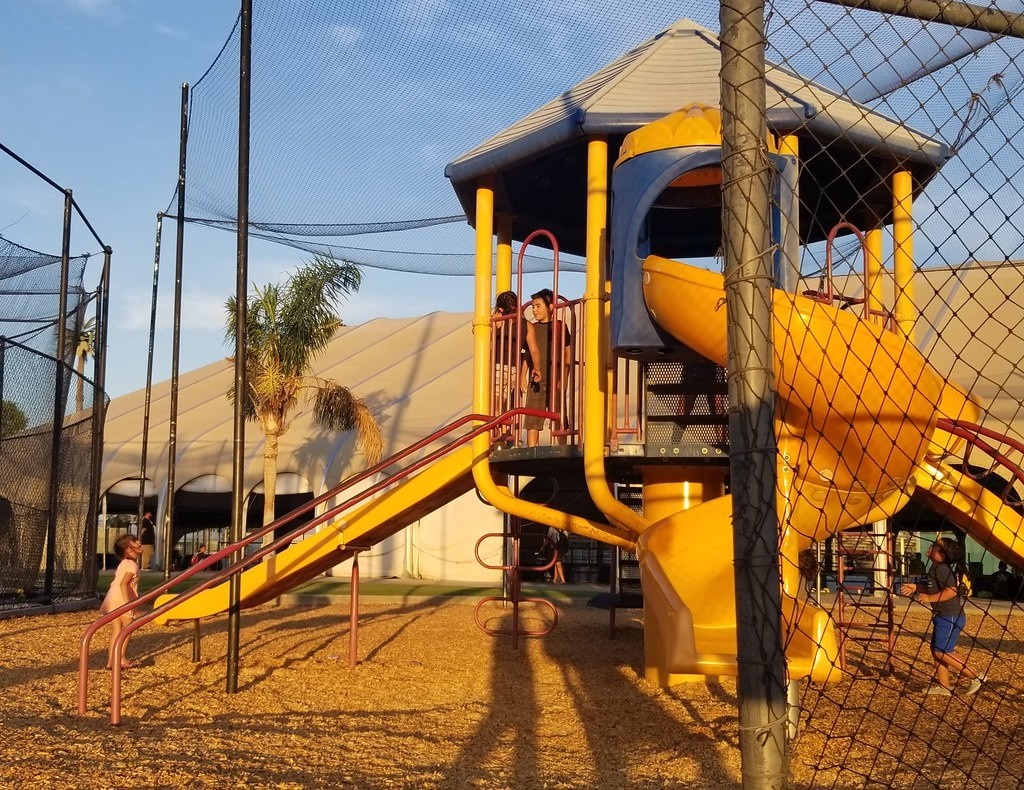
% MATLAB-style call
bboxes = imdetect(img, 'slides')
[149,440,494,625]
[637,250,943,691]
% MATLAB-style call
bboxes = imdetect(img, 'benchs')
[826,575,919,595]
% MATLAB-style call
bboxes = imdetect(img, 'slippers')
[122,659,138,670]
[105,667,112,670]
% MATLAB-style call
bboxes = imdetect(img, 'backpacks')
[191,552,201,565]
[555,528,569,554]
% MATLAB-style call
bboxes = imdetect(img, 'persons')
[540,526,569,584]
[99,535,145,672]
[990,560,1017,601]
[908,552,926,576]
[521,288,571,446]
[798,545,830,594]
[900,538,988,696]
[490,290,542,453]
[139,511,155,571]
[190,545,211,571]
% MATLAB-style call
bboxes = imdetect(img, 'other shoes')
[922,685,950,696]
[966,673,988,695]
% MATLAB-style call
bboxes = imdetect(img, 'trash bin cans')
[968,562,983,577]
